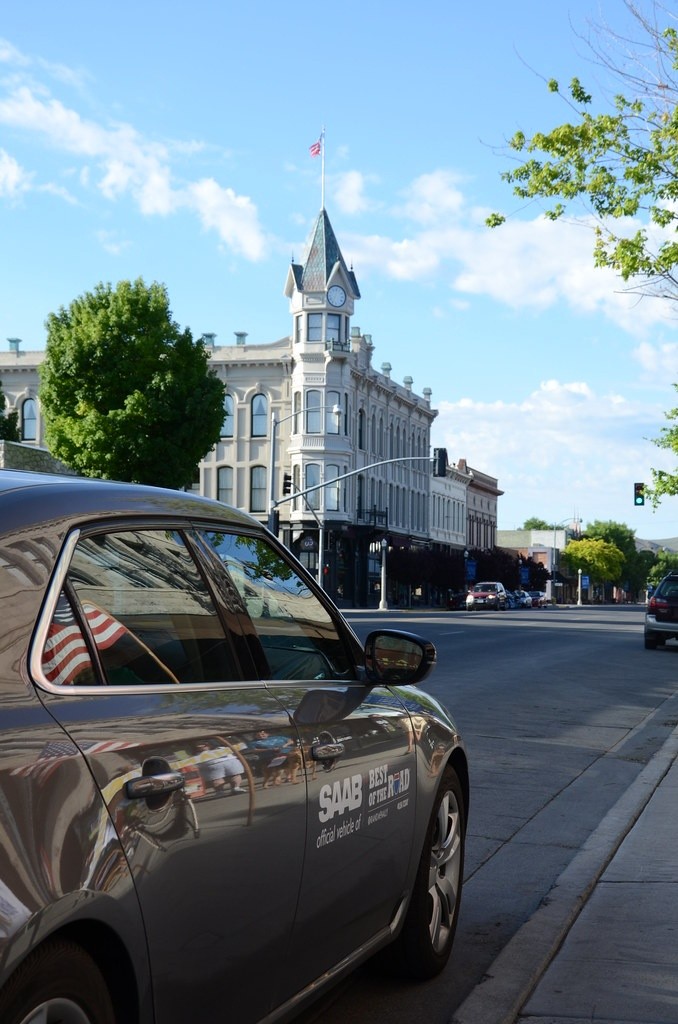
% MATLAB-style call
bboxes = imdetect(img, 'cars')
[465,580,549,612]
[0,466,469,1023]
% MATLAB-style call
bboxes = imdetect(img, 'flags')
[41,586,125,685]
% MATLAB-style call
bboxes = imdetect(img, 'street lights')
[377,538,388,611]
[550,517,584,602]
[267,402,342,536]
[576,569,582,607]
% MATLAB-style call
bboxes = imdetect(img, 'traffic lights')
[283,473,291,495]
[434,448,447,477]
[634,483,646,505]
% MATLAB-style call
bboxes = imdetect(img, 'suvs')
[644,568,677,650]
